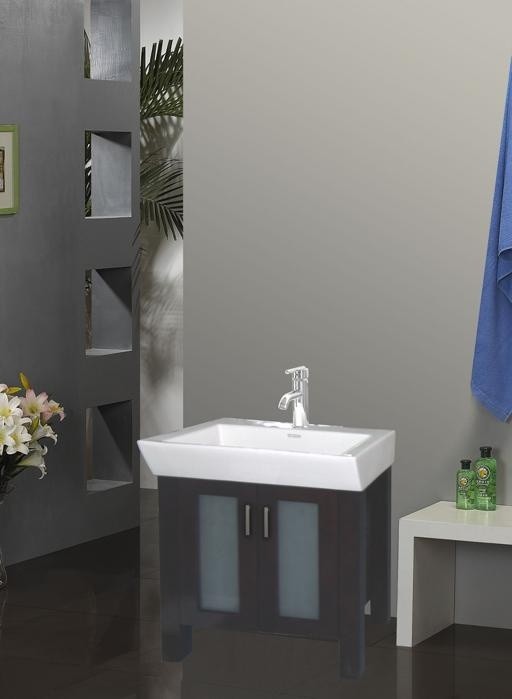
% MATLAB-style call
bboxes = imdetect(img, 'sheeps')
[277,365,310,427]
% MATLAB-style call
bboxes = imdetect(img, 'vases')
[1,372,66,506]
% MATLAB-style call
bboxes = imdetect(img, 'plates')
[158,475,388,679]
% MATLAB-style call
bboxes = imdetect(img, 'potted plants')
[1,123,21,215]
